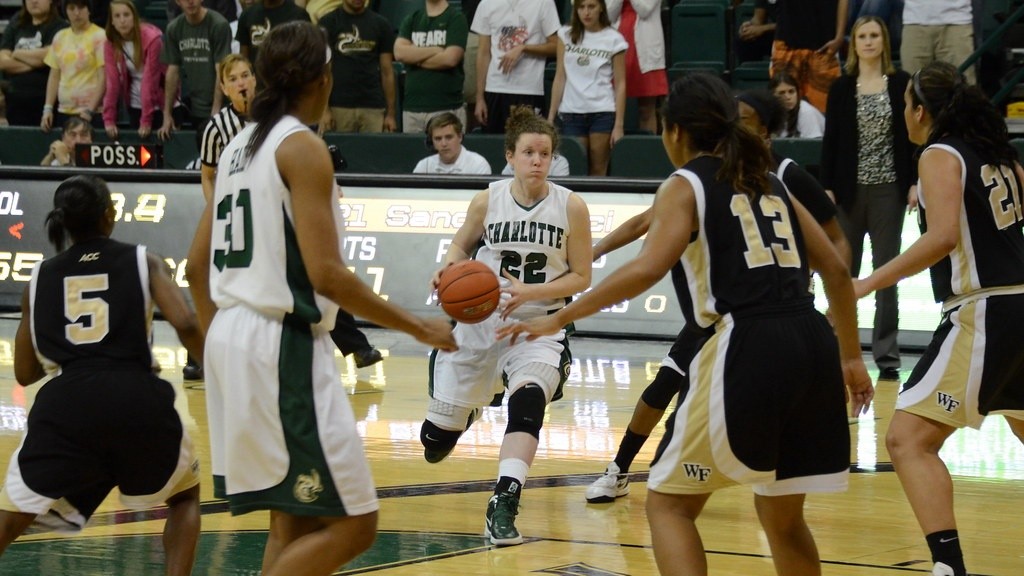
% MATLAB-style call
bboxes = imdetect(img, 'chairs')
[659,0,772,90]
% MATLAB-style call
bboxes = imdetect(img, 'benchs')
[318,130,586,181]
[0,126,203,172]
[607,134,826,179]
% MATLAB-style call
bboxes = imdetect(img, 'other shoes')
[879,368,899,380]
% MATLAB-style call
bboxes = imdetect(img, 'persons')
[0,0,1024,176]
[182,54,384,380]
[821,14,918,379]
[421,105,592,545]
[585,87,852,504]
[851,61,1024,576]
[0,174,207,576]
[496,73,876,576]
[184,20,459,576]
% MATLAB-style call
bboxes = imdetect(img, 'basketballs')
[436,258,502,325]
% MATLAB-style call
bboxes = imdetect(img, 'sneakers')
[425,405,483,464]
[184,363,203,381]
[484,482,523,546]
[584,462,629,503]
[488,547,518,576]
[585,499,630,538]
[353,345,384,368]
